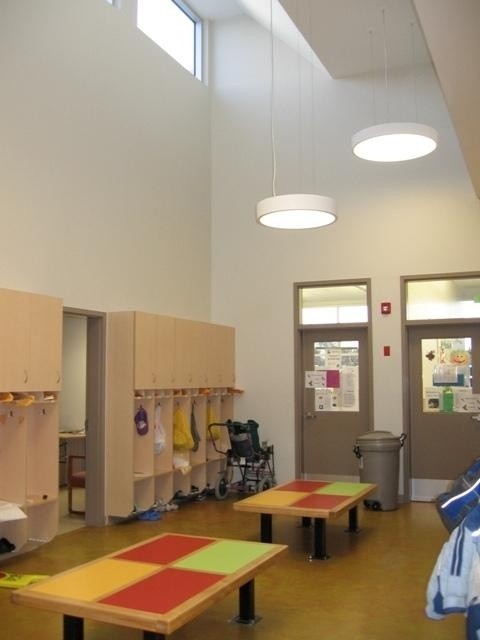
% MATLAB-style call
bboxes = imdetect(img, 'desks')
[232,479,378,560]
[11,532,291,640]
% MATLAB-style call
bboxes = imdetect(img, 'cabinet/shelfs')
[0,286,63,560]
[106,311,236,518]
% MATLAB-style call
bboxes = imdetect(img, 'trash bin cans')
[351,430,407,511]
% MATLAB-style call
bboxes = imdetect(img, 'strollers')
[206,418,278,501]
